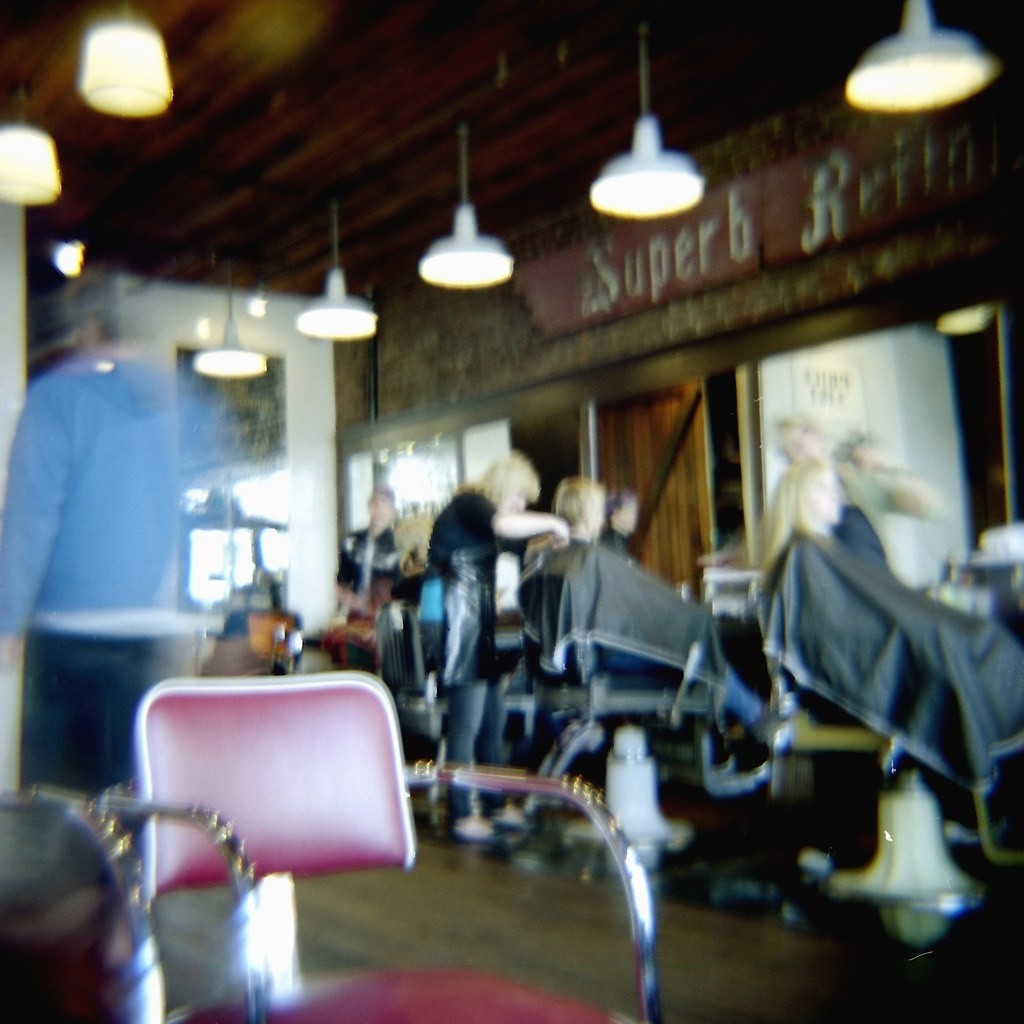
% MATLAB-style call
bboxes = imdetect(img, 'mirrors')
[749,324,973,600]
[177,346,288,608]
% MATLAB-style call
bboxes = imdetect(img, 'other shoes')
[734,739,770,776]
[711,741,732,767]
[493,805,525,828]
[454,817,494,840]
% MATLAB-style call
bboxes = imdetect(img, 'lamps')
[0,88,63,207]
[421,120,515,291]
[190,269,271,378]
[76,1,175,118]
[296,199,380,341]
[843,0,1000,113]
[584,16,711,217]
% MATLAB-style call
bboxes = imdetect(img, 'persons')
[335,486,402,626]
[600,486,640,561]
[523,476,795,755]
[752,435,1007,846]
[418,452,570,844]
[0,268,236,861]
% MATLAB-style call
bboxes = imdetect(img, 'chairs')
[521,539,774,901]
[372,597,540,817]
[93,671,665,1024]
[754,539,1024,950]
